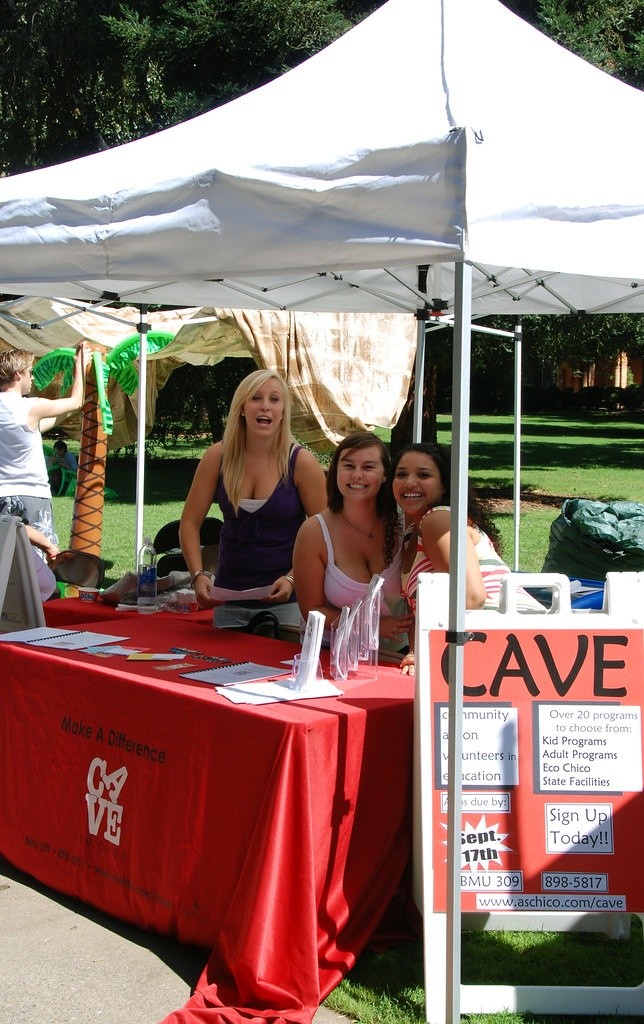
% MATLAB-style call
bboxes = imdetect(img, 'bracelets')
[190,569,213,590]
[409,649,416,654]
[283,575,295,589]
[47,548,57,558]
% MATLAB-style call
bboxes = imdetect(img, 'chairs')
[52,549,104,593]
[152,518,223,581]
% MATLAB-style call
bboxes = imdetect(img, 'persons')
[48,440,77,496]
[0,342,93,563]
[25,523,61,603]
[382,442,548,678]
[178,369,329,646]
[292,430,415,657]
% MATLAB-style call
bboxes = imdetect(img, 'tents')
[0,294,525,577]
[0,0,644,1024]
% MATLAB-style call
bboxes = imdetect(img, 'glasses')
[19,368,33,378]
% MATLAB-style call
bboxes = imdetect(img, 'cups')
[290,654,323,692]
[79,587,99,602]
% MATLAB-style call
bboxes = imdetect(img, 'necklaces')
[340,509,376,537]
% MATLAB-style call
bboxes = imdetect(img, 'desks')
[0,594,414,1024]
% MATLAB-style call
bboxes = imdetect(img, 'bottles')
[137,538,157,614]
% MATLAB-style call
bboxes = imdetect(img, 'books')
[178,661,297,686]
[0,625,131,652]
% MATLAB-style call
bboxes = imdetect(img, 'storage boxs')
[522,578,607,608]
